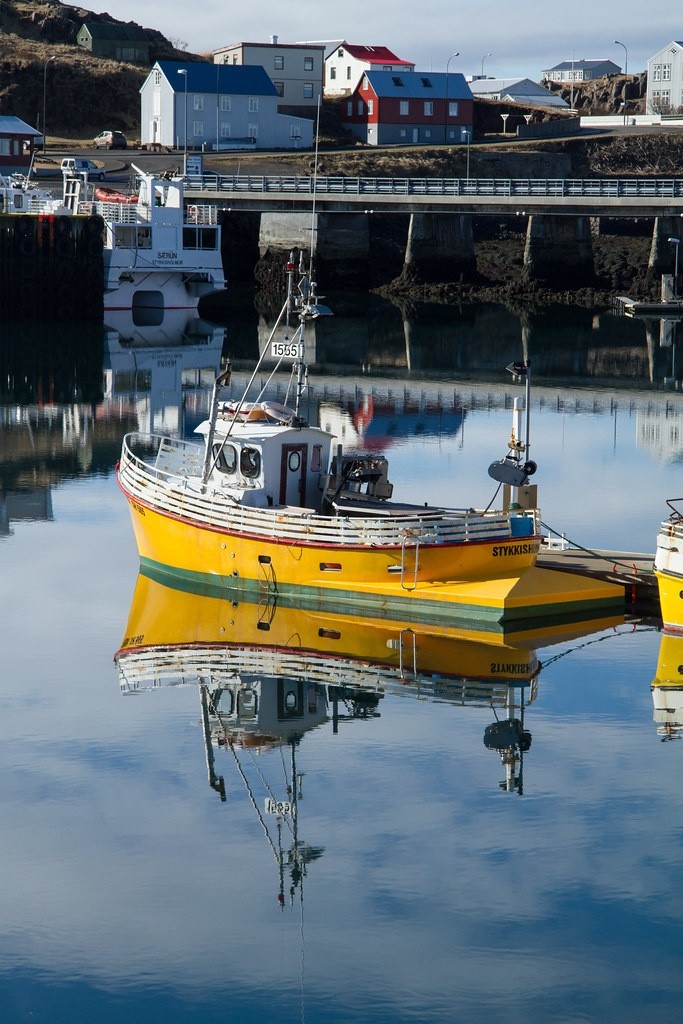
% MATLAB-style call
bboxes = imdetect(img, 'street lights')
[177,68,189,174]
[482,51,492,81]
[667,238,680,296]
[569,48,576,110]
[214,54,231,153]
[614,40,629,124]
[663,323,677,382]
[460,129,471,187]
[444,51,461,145]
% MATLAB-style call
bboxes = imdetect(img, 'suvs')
[92,130,129,150]
[60,159,106,183]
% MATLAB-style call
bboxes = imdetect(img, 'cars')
[22,135,44,150]
[43,55,58,152]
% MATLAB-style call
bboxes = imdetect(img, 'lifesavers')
[260,399,296,422]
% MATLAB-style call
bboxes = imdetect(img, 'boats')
[654,497,683,632]
[649,630,683,743]
[108,562,544,912]
[111,235,634,652]
[95,187,139,204]
